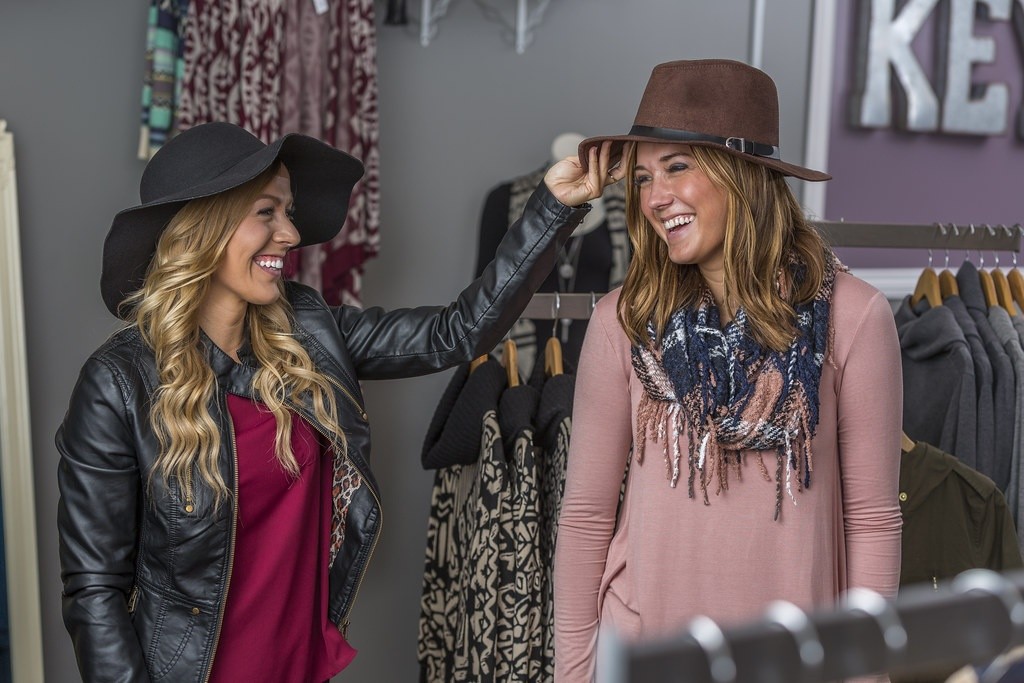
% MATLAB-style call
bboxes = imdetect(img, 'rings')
[607,172,618,184]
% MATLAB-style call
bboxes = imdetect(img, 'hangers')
[464,223,1024,390]
[689,570,1023,683]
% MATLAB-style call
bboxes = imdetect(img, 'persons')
[551,58,903,683]
[54,119,630,683]
[475,135,630,292]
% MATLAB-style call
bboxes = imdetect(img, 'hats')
[100,122,365,322]
[577,58,834,183]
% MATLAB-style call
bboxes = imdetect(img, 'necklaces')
[557,222,585,278]
